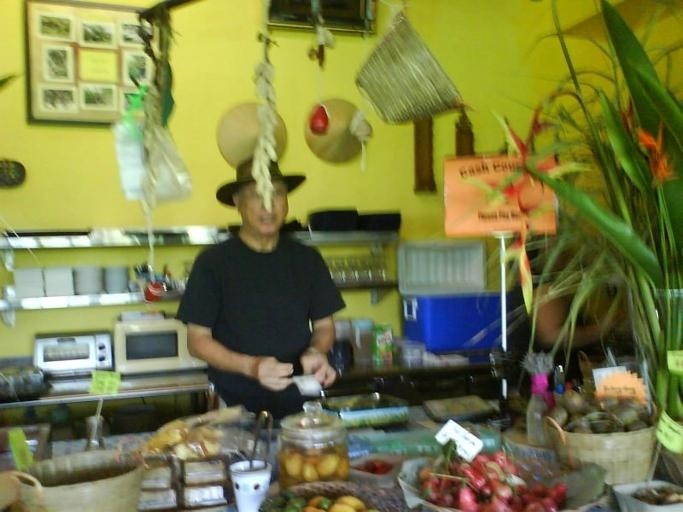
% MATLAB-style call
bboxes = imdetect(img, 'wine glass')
[325,254,387,283]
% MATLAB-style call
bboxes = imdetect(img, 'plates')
[13,265,129,298]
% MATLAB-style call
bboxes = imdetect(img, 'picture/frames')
[24,2,170,129]
[264,2,375,35]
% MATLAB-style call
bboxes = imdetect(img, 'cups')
[229,461,272,512]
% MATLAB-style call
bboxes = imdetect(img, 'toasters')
[34,334,112,377]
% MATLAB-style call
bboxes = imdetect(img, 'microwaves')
[113,317,217,376]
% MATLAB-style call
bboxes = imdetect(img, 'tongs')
[250,410,272,467]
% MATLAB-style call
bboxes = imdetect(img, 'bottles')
[351,320,374,372]
[330,320,351,378]
[526,393,548,446]
[82,415,108,450]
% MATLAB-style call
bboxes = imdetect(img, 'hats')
[216,159,306,207]
[305,99,372,164]
[215,103,287,170]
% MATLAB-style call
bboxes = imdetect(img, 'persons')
[520,286,633,391]
[176,158,346,418]
